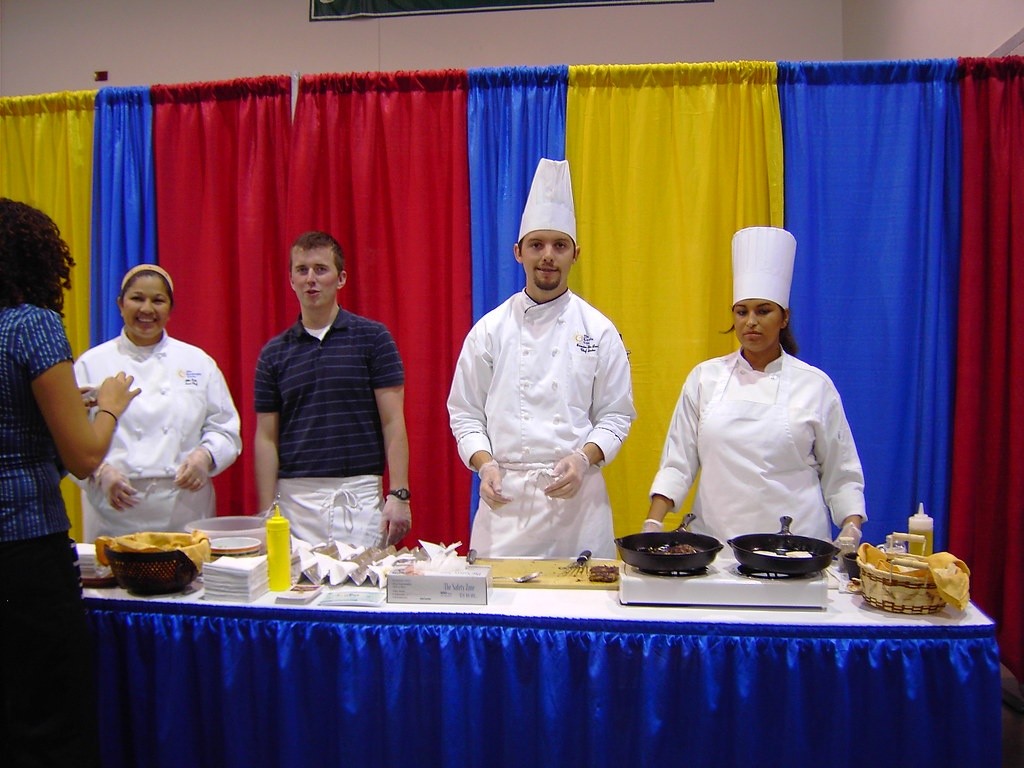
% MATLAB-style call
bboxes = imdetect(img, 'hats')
[517,155,578,254]
[731,227,797,311]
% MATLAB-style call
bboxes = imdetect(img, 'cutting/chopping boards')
[472,559,619,590]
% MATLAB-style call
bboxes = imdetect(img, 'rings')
[196,478,202,486]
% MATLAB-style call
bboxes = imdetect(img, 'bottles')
[838,537,856,573]
[265,504,291,592]
[883,535,907,554]
[907,502,933,558]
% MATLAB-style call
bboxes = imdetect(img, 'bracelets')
[95,410,118,426]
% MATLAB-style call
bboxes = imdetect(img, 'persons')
[253,232,413,551]
[448,159,633,560]
[0,196,141,768]
[643,225,868,562]
[72,262,243,544]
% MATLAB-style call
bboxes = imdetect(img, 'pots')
[614,513,724,571]
[727,516,841,574]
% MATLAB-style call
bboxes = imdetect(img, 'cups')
[842,552,860,581]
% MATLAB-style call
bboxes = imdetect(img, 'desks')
[84,555,1000,768]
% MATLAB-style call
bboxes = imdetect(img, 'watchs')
[387,488,411,500]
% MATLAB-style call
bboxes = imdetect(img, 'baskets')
[101,537,209,597]
[857,531,971,615]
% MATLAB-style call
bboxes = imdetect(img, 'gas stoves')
[619,557,829,610]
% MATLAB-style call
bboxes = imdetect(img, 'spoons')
[492,572,541,583]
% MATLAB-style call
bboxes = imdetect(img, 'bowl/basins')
[210,537,262,563]
[184,515,267,555]
